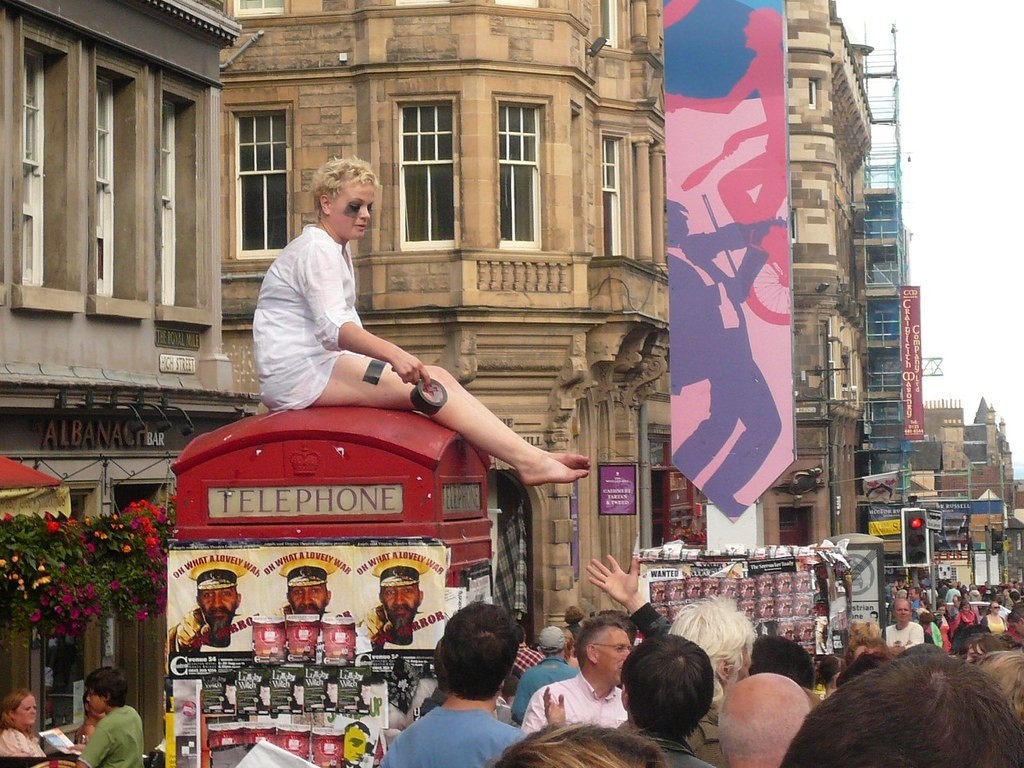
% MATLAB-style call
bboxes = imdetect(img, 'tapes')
[410,378,448,416]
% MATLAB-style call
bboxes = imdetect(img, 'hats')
[370,559,429,588]
[539,626,566,654]
[188,560,249,590]
[278,558,337,588]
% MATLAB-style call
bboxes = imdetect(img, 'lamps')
[99,400,146,432]
[814,282,830,294]
[160,403,195,435]
[135,399,172,431]
[586,36,607,57]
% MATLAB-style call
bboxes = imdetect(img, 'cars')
[944,601,1013,631]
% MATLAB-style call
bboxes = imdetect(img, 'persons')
[277,555,337,616]
[82,693,106,745]
[0,688,48,758]
[370,549,1023,766]
[72,665,145,768]
[250,154,594,487]
[167,561,246,652]
[357,555,424,647]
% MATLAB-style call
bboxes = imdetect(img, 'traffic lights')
[991,528,1004,554]
[900,508,931,568]
